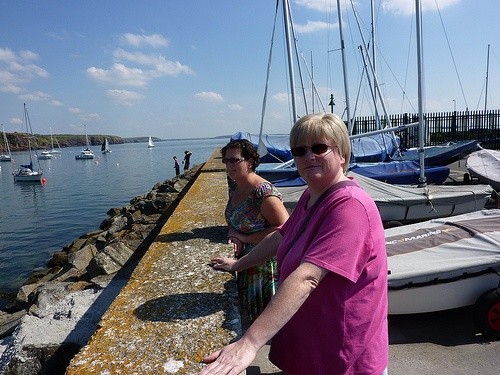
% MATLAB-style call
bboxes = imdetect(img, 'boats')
[39,151,53,159]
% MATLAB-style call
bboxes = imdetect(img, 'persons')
[173,150,192,176]
[198,112,388,375]
[220,139,290,324]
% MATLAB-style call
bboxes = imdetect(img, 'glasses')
[222,158,247,163]
[291,144,338,156]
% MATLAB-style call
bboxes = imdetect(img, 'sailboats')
[0,122,12,161]
[147,134,155,148]
[74,120,95,159]
[101,137,111,154]
[12,102,43,182]
[232,0,500,317]
[44,126,62,153]
